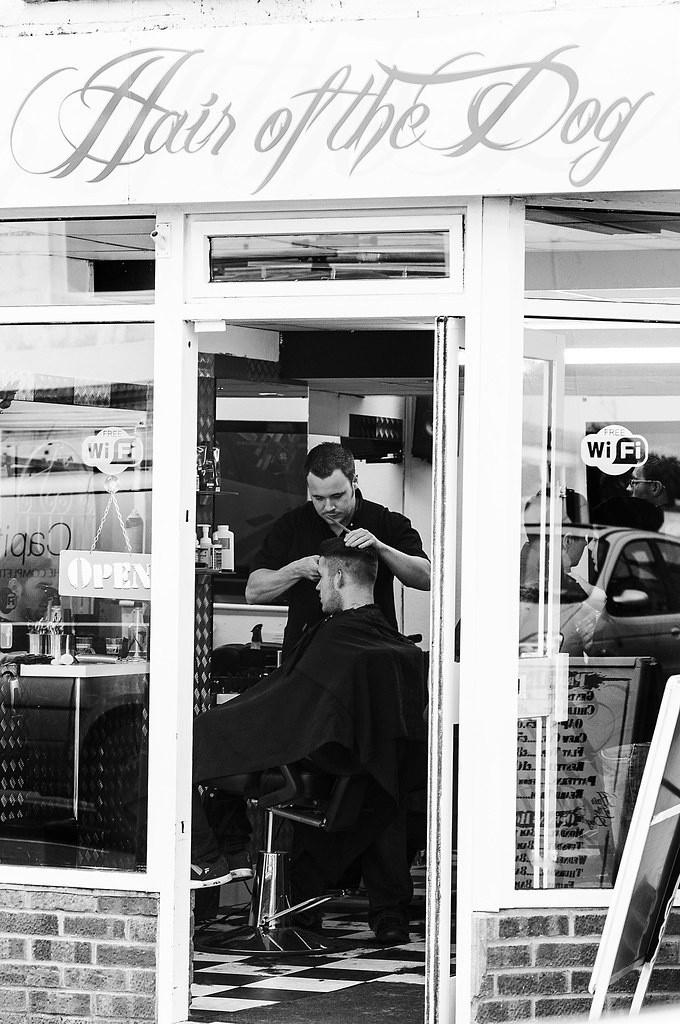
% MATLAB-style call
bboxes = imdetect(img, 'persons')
[585,497,663,603]
[1,533,55,621]
[192,538,391,890]
[244,441,430,669]
[522,487,590,590]
[628,452,679,538]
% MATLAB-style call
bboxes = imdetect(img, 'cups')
[74,636,93,654]
[105,637,123,657]
[42,632,77,662]
[29,633,48,655]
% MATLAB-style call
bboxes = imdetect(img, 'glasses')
[630,479,663,490]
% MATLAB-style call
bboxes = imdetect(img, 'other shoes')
[373,910,411,947]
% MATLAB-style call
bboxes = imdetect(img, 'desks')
[18,658,150,840]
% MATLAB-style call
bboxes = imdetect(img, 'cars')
[520,520,680,675]
[0,658,154,852]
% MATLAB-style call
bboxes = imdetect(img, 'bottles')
[127,607,148,661]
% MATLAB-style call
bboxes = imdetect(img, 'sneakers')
[226,851,254,882]
[190,856,233,890]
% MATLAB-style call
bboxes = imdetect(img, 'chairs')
[196,642,426,956]
